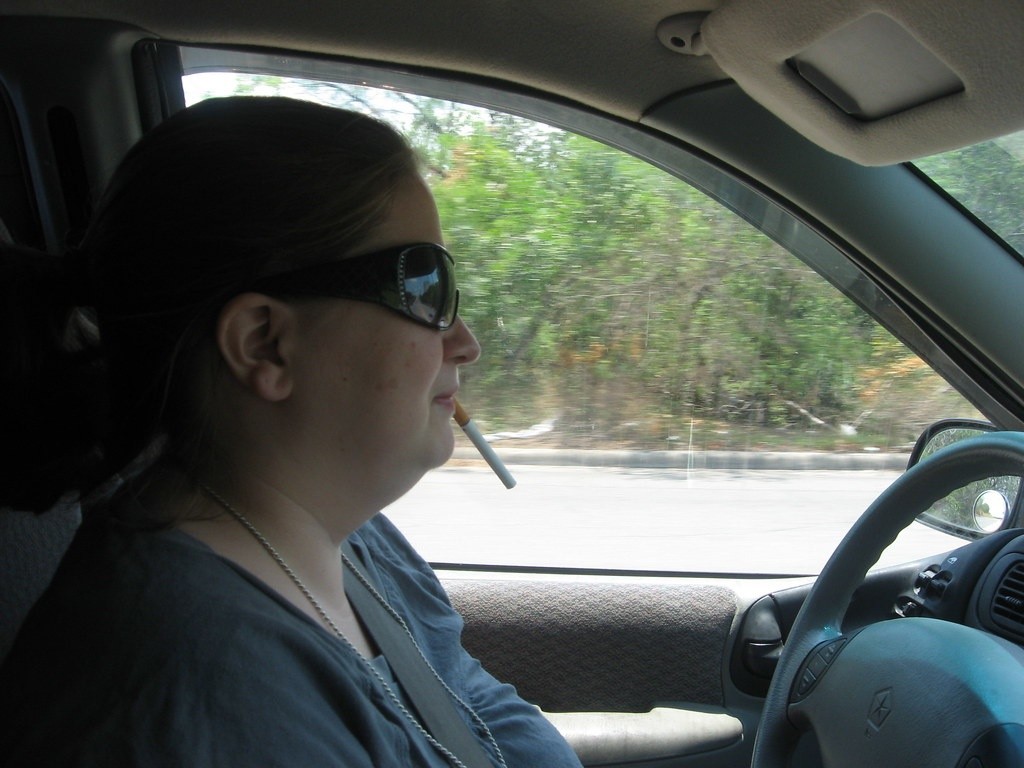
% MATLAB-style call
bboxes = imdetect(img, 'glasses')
[273,242,459,330]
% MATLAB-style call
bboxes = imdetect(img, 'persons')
[0,96,581,768]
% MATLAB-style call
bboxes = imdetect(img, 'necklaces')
[198,482,506,768]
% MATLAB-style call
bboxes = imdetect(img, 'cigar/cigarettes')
[452,395,517,489]
